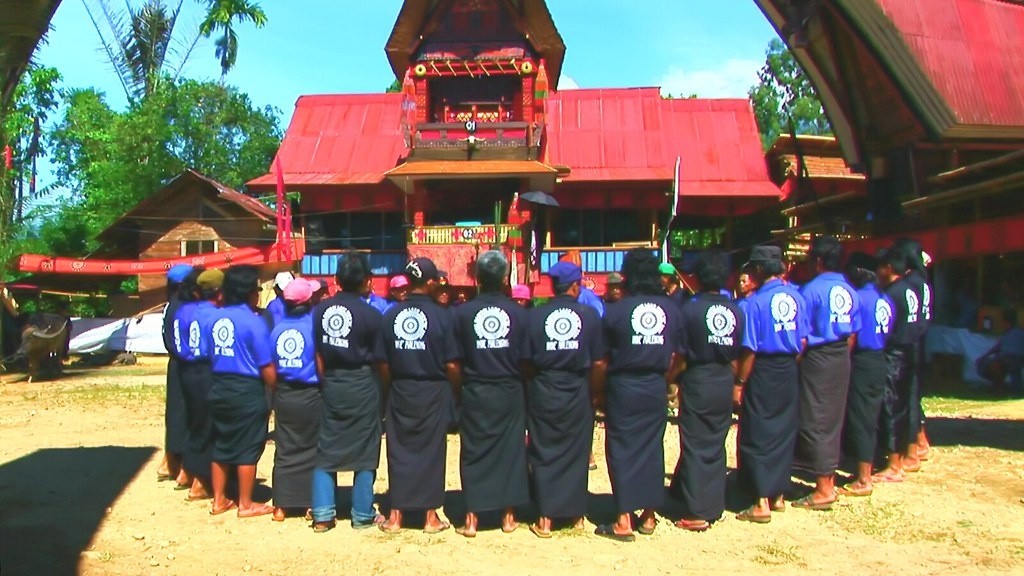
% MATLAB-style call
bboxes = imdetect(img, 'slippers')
[158,443,932,544]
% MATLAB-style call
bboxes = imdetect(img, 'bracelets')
[735,376,748,386]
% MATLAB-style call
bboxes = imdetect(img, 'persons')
[157,235,935,538]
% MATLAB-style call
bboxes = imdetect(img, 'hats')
[549,261,584,282]
[741,245,782,266]
[284,278,321,307]
[405,257,447,284]
[659,263,676,274]
[512,284,530,300]
[196,270,224,291]
[390,276,408,288]
[606,272,624,283]
[166,264,194,285]
[273,271,293,290]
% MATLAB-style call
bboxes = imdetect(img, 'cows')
[6,310,73,383]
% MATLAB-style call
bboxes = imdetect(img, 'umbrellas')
[519,189,560,223]
